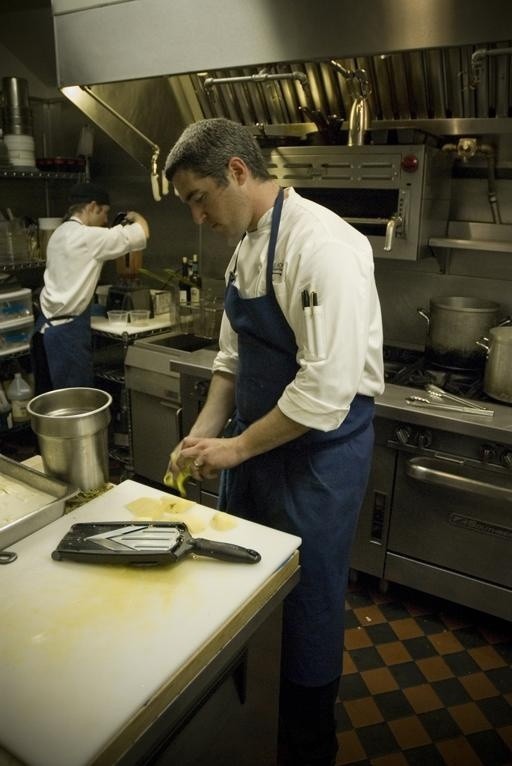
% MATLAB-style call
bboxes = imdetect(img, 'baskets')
[169,300,224,339]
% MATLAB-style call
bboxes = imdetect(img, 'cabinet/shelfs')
[0,163,90,270]
[91,314,197,474]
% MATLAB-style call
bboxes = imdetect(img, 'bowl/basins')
[106,309,129,328]
[127,308,151,329]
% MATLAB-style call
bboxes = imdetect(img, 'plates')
[3,132,35,168]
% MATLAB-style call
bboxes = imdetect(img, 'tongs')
[408,381,498,420]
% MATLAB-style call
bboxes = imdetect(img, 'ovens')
[249,141,454,268]
[383,442,512,623]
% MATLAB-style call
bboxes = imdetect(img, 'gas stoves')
[398,355,512,407]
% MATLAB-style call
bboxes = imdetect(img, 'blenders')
[103,210,156,321]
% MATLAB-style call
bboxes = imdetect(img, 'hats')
[72,182,111,204]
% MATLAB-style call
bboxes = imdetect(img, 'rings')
[192,460,204,468]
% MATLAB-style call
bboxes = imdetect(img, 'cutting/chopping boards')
[2,478,306,759]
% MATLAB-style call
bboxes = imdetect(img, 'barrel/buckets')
[27,385,112,493]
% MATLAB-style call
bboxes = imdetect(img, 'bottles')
[179,255,190,309]
[189,251,201,309]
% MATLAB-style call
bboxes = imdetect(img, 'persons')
[29,186,153,392]
[163,117,387,765]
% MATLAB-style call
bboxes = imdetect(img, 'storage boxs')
[0,321,37,355]
[0,287,37,325]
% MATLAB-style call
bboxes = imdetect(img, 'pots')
[417,290,512,373]
[473,318,512,404]
